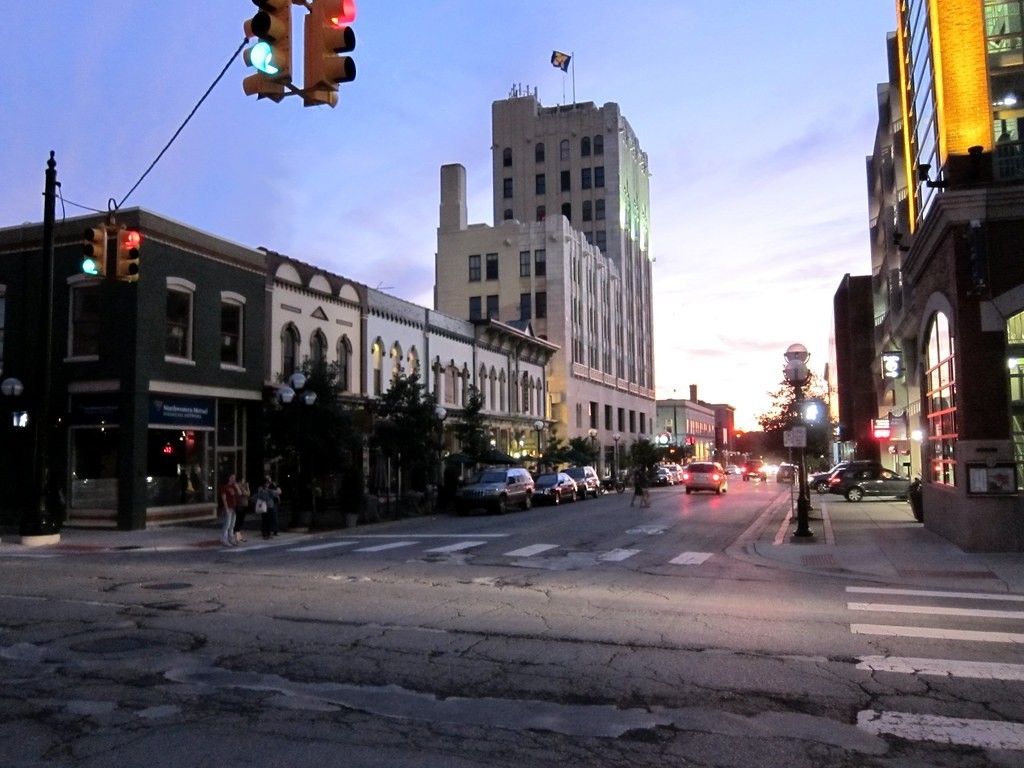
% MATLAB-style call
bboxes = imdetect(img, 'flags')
[551,50,572,73]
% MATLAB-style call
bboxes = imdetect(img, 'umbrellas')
[477,449,517,464]
[436,454,476,464]
[518,451,595,464]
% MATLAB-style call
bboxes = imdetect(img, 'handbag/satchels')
[236,495,248,508]
[254,497,268,515]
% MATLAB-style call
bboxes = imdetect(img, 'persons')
[234,473,250,543]
[258,479,274,540]
[366,471,382,524]
[221,471,243,547]
[264,473,282,536]
[628,466,657,508]
[443,463,462,509]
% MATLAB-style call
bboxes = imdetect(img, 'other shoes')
[233,542,239,546]
[262,535,270,540]
[241,538,248,542]
[273,532,280,536]
[223,543,233,547]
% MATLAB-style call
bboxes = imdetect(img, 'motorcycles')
[598,473,625,495]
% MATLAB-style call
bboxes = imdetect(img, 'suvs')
[455,467,536,515]
[807,460,884,494]
[558,465,601,500]
[742,459,768,482]
[765,463,800,484]
[684,462,729,495]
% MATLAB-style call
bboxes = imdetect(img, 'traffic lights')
[249,0,293,83]
[303,1,357,109]
[81,222,108,280]
[243,17,286,103]
[115,228,142,282]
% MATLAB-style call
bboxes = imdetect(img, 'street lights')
[783,359,815,538]
[433,406,447,514]
[589,428,597,474]
[613,432,622,482]
[783,343,814,511]
[533,420,545,474]
[0,377,24,527]
[275,373,318,529]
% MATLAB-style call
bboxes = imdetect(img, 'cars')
[646,464,686,486]
[827,464,916,503]
[725,464,744,475]
[532,472,579,505]
